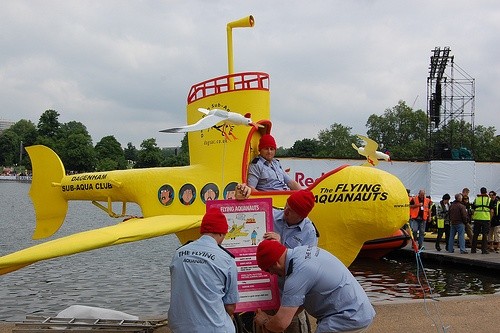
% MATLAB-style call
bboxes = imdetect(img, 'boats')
[363,228,410,249]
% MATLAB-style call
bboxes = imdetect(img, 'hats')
[287,190,315,218]
[200,209,229,233]
[258,134,277,150]
[256,240,286,271]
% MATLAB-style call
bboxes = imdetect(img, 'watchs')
[262,316,270,328]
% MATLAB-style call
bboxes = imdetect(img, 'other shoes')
[489,247,494,251]
[446,246,449,251]
[472,250,477,253]
[435,246,441,252]
[482,250,489,254]
[460,250,468,254]
[496,248,499,252]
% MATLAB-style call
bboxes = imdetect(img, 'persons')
[167,207,241,333]
[235,182,320,333]
[405,187,500,255]
[247,134,303,191]
[254,239,377,333]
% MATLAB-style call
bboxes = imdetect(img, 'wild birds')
[351,134,391,167]
[159,107,259,143]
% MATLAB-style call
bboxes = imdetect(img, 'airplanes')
[0,15,412,280]
